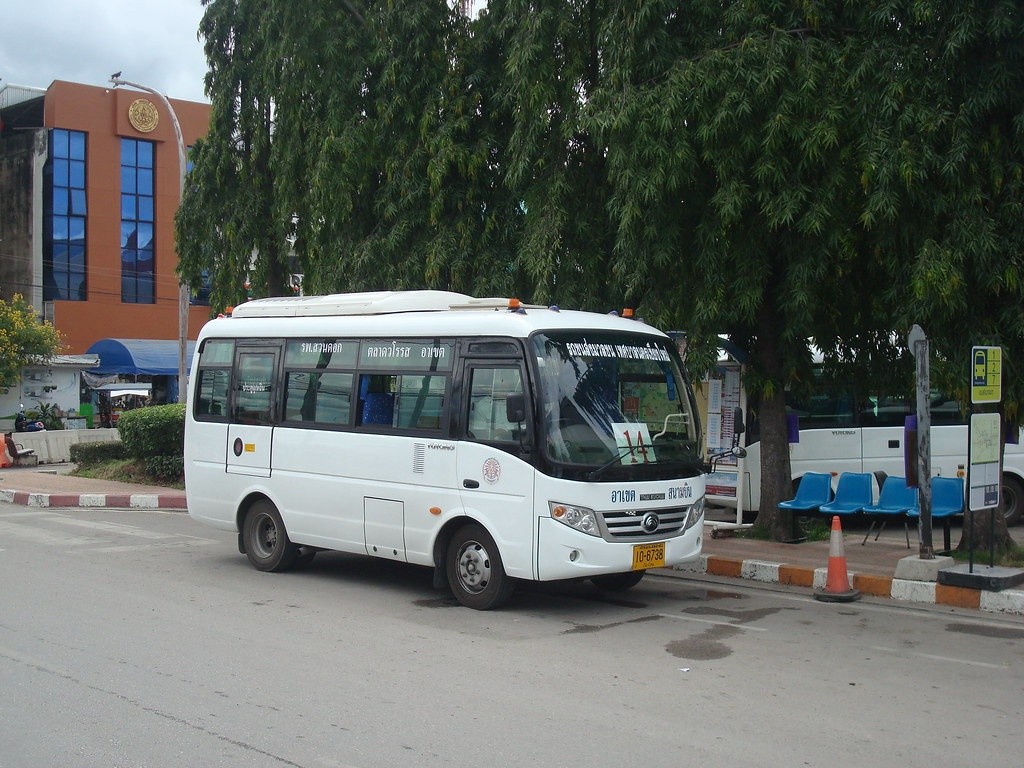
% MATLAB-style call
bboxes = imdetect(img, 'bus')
[670,330,1024,528]
[185,291,706,611]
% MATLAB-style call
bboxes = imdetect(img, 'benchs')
[778,472,967,557]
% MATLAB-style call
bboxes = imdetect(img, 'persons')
[98,392,110,421]
[474,375,528,440]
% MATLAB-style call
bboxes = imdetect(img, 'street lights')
[109,75,188,404]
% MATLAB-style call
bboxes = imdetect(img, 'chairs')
[7,436,39,466]
[362,393,394,427]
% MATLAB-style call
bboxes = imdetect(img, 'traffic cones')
[814,517,861,602]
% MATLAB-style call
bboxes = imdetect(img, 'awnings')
[85,338,196,409]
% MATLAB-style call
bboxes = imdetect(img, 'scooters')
[15,403,46,432]
